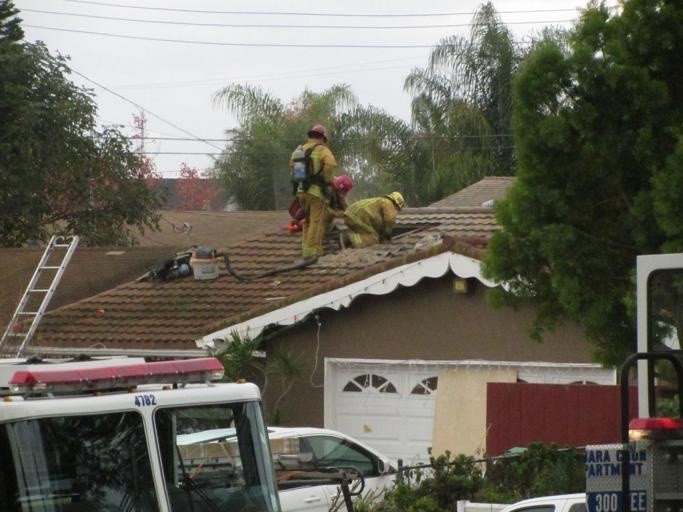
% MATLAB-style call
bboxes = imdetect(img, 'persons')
[336,191,405,253]
[287,124,338,261]
[287,174,354,234]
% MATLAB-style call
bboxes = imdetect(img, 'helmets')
[308,125,328,142]
[333,175,353,195]
[387,191,405,210]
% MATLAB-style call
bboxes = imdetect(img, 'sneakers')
[338,232,346,251]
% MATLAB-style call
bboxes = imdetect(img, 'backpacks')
[290,143,325,185]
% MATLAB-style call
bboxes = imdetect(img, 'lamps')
[315,314,327,329]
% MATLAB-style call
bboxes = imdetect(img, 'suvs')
[172,423,427,512]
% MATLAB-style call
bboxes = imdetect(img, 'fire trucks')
[0,355,282,512]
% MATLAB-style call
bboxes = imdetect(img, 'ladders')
[0,235,81,359]
